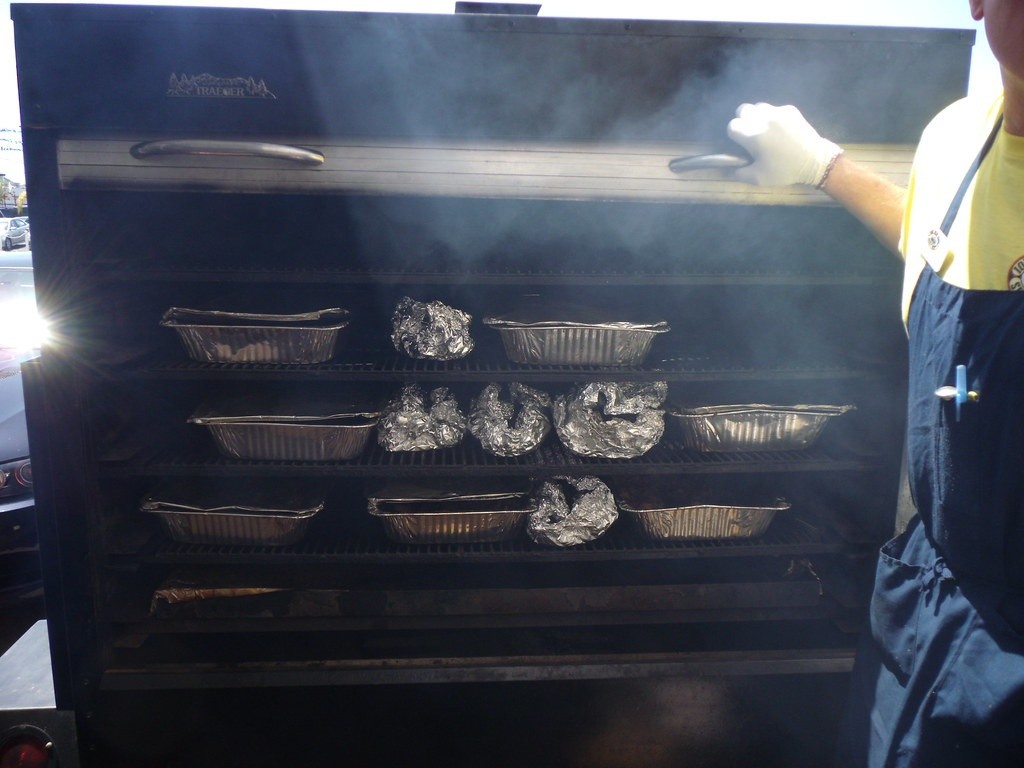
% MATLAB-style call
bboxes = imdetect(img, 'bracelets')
[818,148,844,190]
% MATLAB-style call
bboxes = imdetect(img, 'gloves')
[725,102,845,190]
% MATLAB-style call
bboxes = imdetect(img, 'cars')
[0,247,49,556]
[0,215,32,251]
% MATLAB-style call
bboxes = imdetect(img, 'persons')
[720,0,1023,768]
